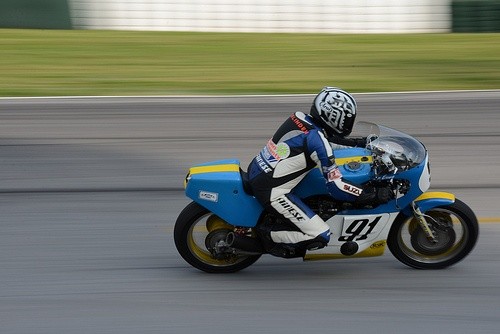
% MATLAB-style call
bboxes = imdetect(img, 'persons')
[210,87,395,259]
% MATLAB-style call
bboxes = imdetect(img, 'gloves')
[360,179,395,206]
[356,136,378,148]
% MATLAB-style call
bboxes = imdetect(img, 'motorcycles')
[172,120,480,271]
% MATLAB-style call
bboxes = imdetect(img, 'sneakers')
[260,226,273,253]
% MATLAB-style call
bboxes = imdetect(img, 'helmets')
[310,87,357,137]
